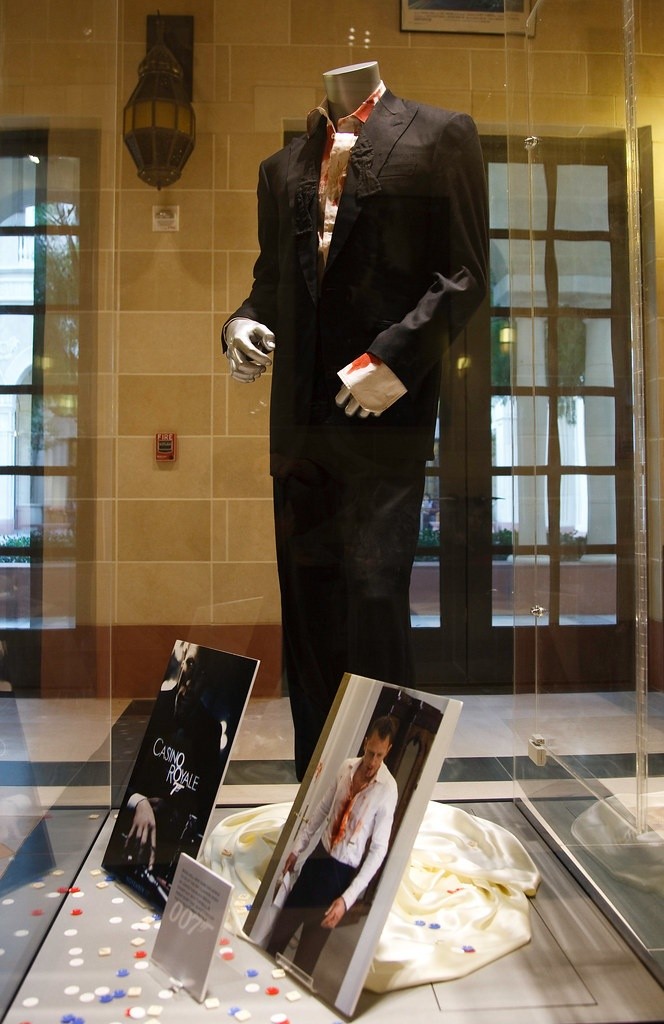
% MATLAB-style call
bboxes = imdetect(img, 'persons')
[107,645,223,871]
[221,59,487,783]
[265,717,398,982]
[421,493,439,538]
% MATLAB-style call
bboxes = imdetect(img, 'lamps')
[123,14,194,199]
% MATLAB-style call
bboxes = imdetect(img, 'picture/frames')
[400,0,538,40]
